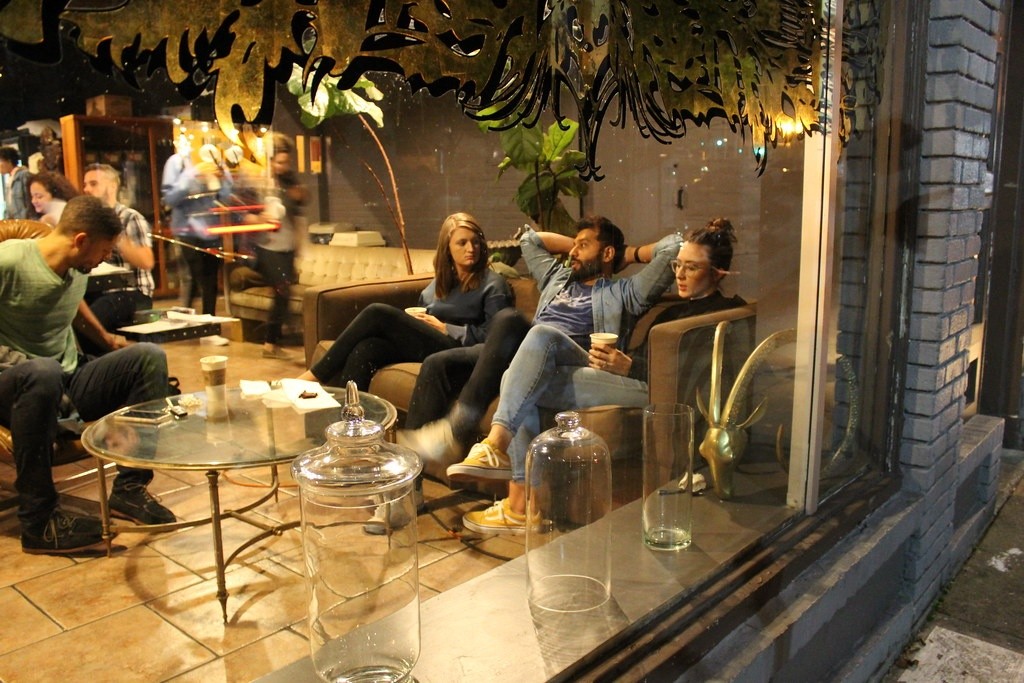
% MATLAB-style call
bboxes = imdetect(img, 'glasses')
[670,260,713,277]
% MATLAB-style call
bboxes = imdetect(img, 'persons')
[229,133,308,361]
[263,213,515,452]
[0,152,176,553]
[366,211,684,533]
[446,218,748,536]
[160,136,232,346]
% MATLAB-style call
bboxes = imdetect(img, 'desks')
[81,382,398,627]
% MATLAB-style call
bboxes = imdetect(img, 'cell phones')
[114,410,170,423]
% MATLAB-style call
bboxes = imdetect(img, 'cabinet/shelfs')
[61,114,222,299]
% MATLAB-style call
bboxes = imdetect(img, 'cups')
[200,355,228,394]
[405,306,427,315]
[206,403,233,443]
[589,333,619,354]
[640,403,693,551]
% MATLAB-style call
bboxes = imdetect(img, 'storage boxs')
[86,94,132,115]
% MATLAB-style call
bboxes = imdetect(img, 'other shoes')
[362,485,425,533]
[199,334,229,345]
[396,418,463,465]
[261,346,294,360]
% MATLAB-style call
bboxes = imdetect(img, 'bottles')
[525,411,613,612]
[293,381,423,683]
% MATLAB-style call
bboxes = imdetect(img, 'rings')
[602,361,608,369]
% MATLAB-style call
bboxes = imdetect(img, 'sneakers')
[446,438,513,483]
[21,504,118,553]
[108,482,176,525]
[462,497,544,533]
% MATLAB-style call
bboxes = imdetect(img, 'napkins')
[239,378,340,410]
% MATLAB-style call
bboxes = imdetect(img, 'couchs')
[303,271,759,523]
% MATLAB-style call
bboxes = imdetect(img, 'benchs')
[223,244,440,342]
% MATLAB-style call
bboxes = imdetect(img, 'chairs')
[0,219,104,515]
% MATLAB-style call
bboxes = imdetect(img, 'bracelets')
[634,246,641,263]
